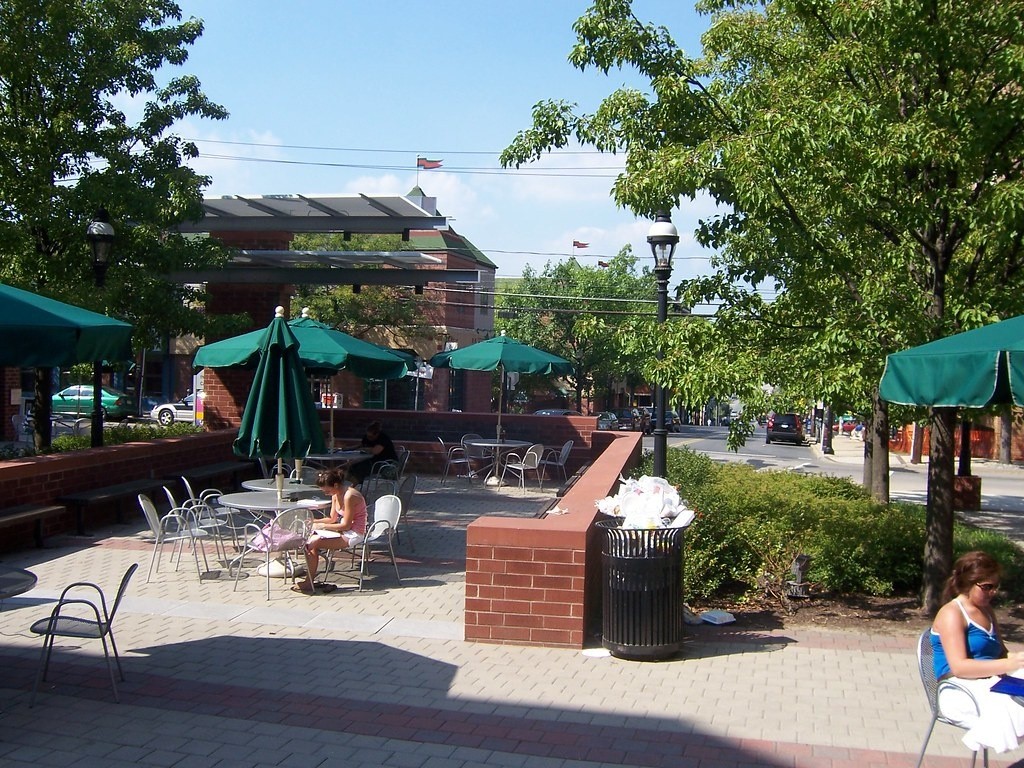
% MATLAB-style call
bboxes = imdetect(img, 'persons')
[707,419,711,426]
[293,469,368,591]
[930,551,1024,754]
[348,421,400,489]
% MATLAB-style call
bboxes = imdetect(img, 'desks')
[302,451,374,479]
[241,479,352,555]
[215,491,334,578]
[50,414,63,435]
[462,439,533,486]
[0,565,38,599]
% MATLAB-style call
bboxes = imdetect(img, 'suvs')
[766,413,802,446]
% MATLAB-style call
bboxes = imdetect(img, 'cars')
[150,393,194,427]
[832,419,855,432]
[52,383,136,421]
[589,411,619,431]
[534,408,584,417]
[610,406,681,433]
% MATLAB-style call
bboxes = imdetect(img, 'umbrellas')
[429,330,570,475]
[876,314,1024,408]
[233,306,328,497]
[0,284,133,368]
[193,307,418,482]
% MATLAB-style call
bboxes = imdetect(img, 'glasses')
[975,583,1000,591]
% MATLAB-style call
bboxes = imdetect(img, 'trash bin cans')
[594,517,691,660]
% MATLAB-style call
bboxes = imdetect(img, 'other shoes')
[323,585,337,594]
[314,582,330,588]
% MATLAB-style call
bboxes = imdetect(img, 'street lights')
[646,210,681,481]
[86,213,117,449]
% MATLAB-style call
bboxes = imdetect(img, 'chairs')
[916,626,988,768]
[24,562,139,709]
[12,412,129,447]
[137,431,574,600]
